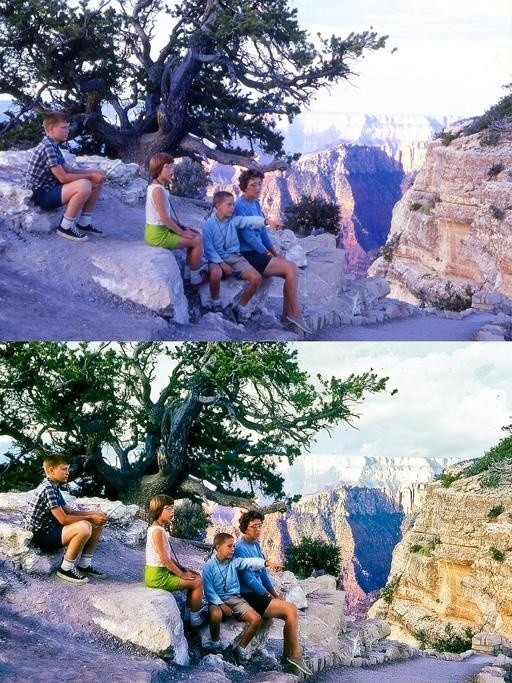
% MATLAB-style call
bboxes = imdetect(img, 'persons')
[143,151,210,295]
[232,168,313,334]
[200,191,286,329]
[143,493,209,630]
[24,453,107,583]
[201,532,286,669]
[27,112,109,242]
[233,509,314,676]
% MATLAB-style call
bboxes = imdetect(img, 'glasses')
[164,506,175,510]
[246,523,262,528]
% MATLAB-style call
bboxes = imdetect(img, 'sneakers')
[184,612,209,635]
[232,303,248,328]
[214,646,224,657]
[287,312,313,335]
[56,567,90,583]
[213,300,222,312]
[184,269,209,291]
[56,225,89,241]
[76,223,106,237]
[287,657,313,675]
[76,563,107,579]
[232,647,245,669]
